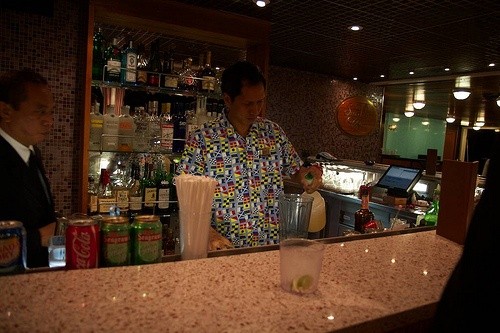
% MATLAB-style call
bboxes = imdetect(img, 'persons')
[1,67,56,267]
[173,62,323,249]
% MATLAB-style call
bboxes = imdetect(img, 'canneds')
[0,220,27,275]
[97,217,134,269]
[65,219,101,270]
[129,215,163,266]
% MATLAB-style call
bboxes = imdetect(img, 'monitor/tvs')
[377,164,423,191]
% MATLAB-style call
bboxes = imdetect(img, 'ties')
[28,150,50,205]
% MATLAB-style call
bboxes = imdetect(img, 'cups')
[417,200,424,205]
[177,210,214,261]
[355,189,375,234]
[278,228,326,295]
[278,194,314,240]
[420,201,428,206]
[390,218,407,229]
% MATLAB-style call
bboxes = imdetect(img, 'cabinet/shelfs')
[71,0,272,217]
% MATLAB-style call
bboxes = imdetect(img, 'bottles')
[301,171,326,232]
[164,228,175,255]
[420,199,438,227]
[87,86,218,153]
[433,183,441,215]
[162,224,169,250]
[86,153,181,218]
[91,27,228,95]
[411,192,417,204]
[361,219,385,234]
[175,237,182,255]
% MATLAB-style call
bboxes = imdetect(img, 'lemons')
[290,274,313,292]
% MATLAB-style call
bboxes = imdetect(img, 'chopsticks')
[174,176,218,212]
